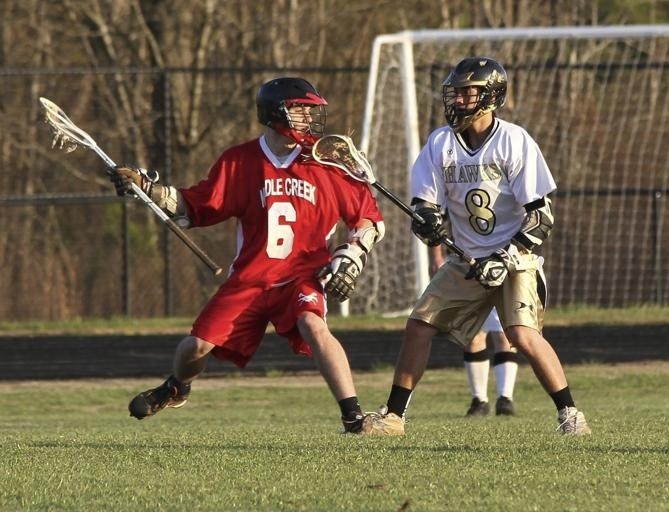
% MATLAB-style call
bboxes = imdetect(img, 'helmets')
[440,56,509,133]
[255,76,329,152]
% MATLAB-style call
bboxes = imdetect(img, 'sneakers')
[554,405,592,436]
[466,396,489,417]
[360,403,406,437]
[129,373,192,421]
[495,395,515,415]
[339,415,365,433]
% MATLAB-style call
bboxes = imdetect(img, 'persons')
[106,76,386,436]
[425,235,519,419]
[362,57,592,441]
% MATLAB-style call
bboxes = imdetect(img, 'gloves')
[103,164,163,204]
[312,240,368,303]
[463,242,527,290]
[411,207,449,248]
